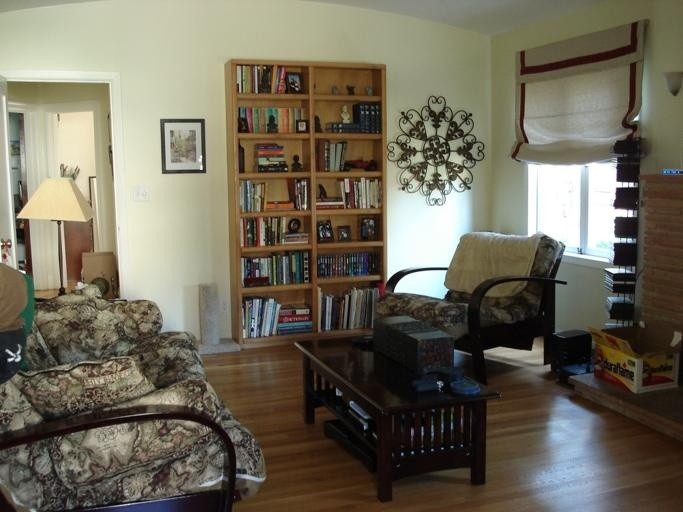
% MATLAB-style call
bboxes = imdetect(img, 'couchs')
[0,298,267,512]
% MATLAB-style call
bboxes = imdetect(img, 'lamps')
[661,72,683,96]
[16,178,96,296]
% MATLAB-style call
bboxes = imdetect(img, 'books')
[240,251,310,288]
[254,143,289,173]
[238,179,267,212]
[316,177,382,210]
[326,103,382,133]
[317,250,382,279]
[239,216,309,248]
[358,215,378,241]
[239,106,308,133]
[240,295,313,338]
[265,201,296,211]
[315,138,379,172]
[316,220,334,243]
[337,225,352,242]
[236,65,306,94]
[294,178,310,211]
[317,283,382,333]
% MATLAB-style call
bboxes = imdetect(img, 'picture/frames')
[285,71,302,93]
[297,120,308,133]
[317,218,377,243]
[160,119,206,173]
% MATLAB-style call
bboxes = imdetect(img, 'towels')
[444,232,544,298]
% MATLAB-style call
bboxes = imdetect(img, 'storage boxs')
[587,317,683,395]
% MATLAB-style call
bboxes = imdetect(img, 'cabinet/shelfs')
[224,58,386,351]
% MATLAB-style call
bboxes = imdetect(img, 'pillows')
[9,300,219,489]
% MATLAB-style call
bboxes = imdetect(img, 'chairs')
[374,232,568,387]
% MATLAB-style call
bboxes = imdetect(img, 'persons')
[291,155,301,169]
